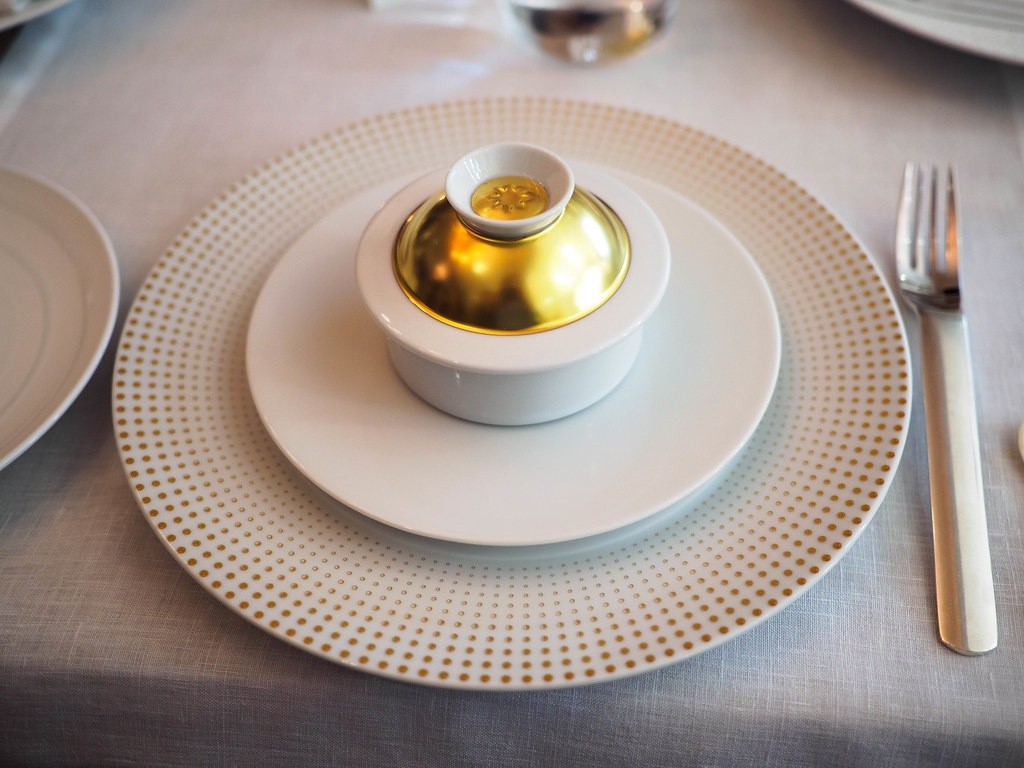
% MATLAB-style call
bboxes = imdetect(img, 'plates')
[0,167,122,471]
[111,97,913,692]
[856,0,1024,64]
[245,171,782,547]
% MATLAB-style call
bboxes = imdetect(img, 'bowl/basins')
[356,141,671,427]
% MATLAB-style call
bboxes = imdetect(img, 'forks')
[897,162,998,654]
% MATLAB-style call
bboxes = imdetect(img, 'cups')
[511,0,666,64]
